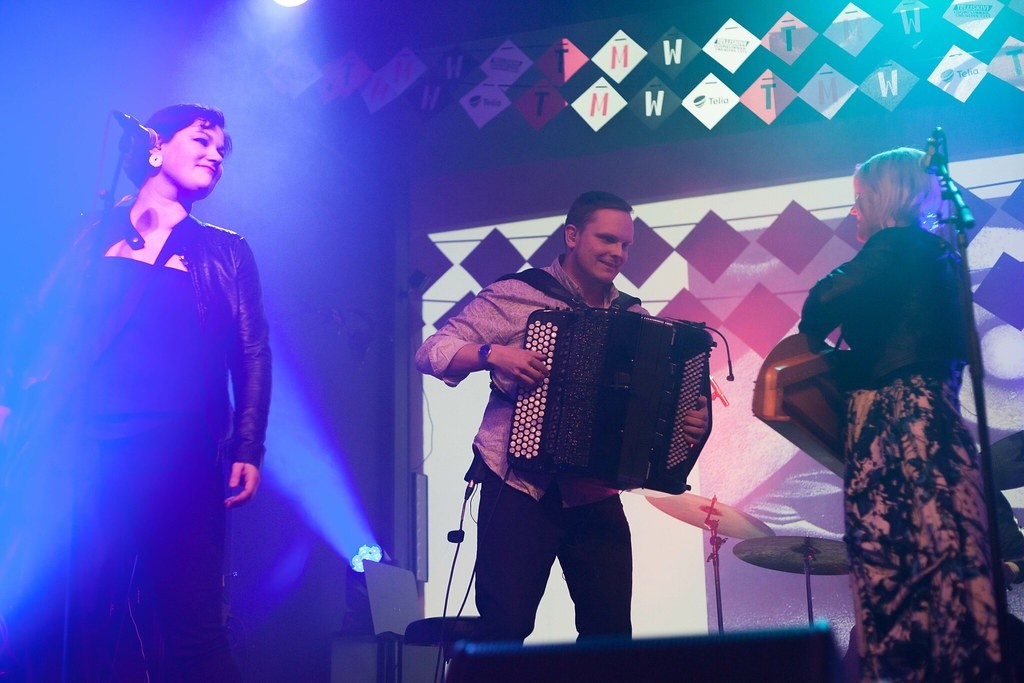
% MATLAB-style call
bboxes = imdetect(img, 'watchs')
[478,343,496,371]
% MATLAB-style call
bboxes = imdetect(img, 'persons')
[48,103,273,683]
[798,147,1024,683]
[414,191,709,641]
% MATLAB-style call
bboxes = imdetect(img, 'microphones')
[920,127,941,174]
[710,375,729,407]
[111,110,158,148]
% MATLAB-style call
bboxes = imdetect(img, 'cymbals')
[733,533,850,575]
[979,429,1024,490]
[643,486,777,538]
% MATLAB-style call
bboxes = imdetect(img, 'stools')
[404,616,478,682]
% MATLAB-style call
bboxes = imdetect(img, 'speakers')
[447,623,848,683]
